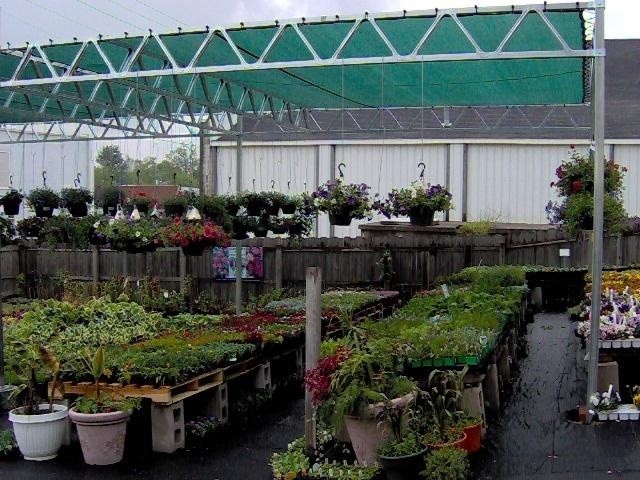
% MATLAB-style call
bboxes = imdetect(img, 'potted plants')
[2,334,69,461]
[0,266,283,453]
[263,266,530,480]
[566,193,627,240]
[69,347,140,465]
[0,187,306,257]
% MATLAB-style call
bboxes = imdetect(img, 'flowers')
[372,177,455,218]
[213,247,263,281]
[543,140,627,223]
[577,268,640,339]
[626,385,640,409]
[589,383,622,410]
[313,176,374,219]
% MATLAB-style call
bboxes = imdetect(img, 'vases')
[597,340,640,348]
[410,206,434,225]
[328,210,351,225]
[597,403,640,421]
